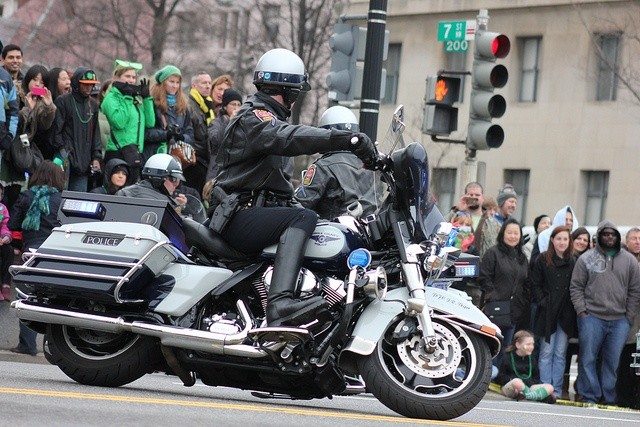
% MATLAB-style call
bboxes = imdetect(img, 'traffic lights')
[327,23,389,101]
[421,76,461,136]
[467,32,511,150]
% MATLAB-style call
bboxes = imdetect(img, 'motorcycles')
[8,104,504,419]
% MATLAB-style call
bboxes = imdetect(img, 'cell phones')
[466,198,479,205]
[32,87,46,96]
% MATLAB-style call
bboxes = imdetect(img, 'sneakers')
[510,388,519,398]
[1,284,11,300]
[545,394,557,402]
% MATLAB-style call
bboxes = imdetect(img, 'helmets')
[252,47,311,104]
[317,105,360,133]
[142,151,186,182]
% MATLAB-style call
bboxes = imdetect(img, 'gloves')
[169,124,182,134]
[329,127,374,162]
[3,134,12,154]
[122,82,134,96]
[137,77,150,97]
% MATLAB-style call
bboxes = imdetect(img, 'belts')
[240,194,292,207]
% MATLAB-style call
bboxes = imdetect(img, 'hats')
[222,87,242,106]
[534,215,549,232]
[113,164,129,174]
[154,65,181,83]
[496,183,517,207]
[79,71,101,84]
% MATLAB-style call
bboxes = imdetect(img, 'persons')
[626,227,640,253]
[499,329,557,404]
[533,225,570,398]
[481,184,518,253]
[556,208,574,226]
[7,159,64,252]
[0,41,19,159]
[294,105,384,221]
[207,47,376,326]
[50,66,102,190]
[473,193,498,254]
[48,67,71,94]
[18,64,57,141]
[187,70,216,170]
[534,214,551,233]
[208,88,242,170]
[115,153,188,209]
[101,59,156,181]
[480,219,532,328]
[572,226,590,252]
[570,218,639,403]
[211,74,234,104]
[144,65,195,160]
[0,183,14,301]
[441,182,485,229]
[2,44,22,79]
[89,157,130,193]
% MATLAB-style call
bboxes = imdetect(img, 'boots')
[266,227,328,327]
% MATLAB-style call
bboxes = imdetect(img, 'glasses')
[601,231,618,237]
[164,174,177,182]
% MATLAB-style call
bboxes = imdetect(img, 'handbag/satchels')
[169,140,196,169]
[12,136,33,169]
[485,299,512,330]
[121,143,145,168]
